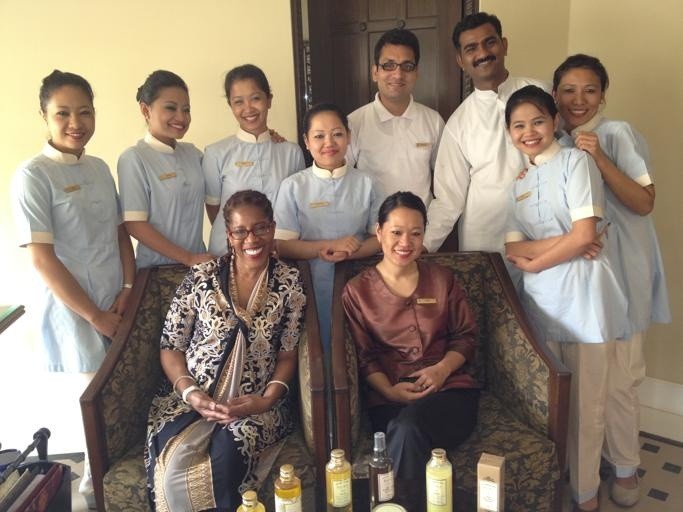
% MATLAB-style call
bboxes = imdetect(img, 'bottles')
[425,447,453,512]
[326,448,353,512]
[273,463,301,512]
[369,431,394,510]
[235,490,266,511]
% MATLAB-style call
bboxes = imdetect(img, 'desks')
[348,479,483,512]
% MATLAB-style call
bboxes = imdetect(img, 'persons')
[343,28,443,228]
[142,189,305,511]
[117,70,289,274]
[201,64,304,260]
[550,54,670,511]
[339,190,484,511]
[11,70,135,511]
[499,86,618,511]
[273,102,384,441]
[418,11,561,258]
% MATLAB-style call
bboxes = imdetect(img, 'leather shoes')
[574,490,602,512]
[610,470,640,508]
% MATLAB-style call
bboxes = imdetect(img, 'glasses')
[226,222,272,240]
[376,59,417,71]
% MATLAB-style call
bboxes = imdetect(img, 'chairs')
[77,256,334,512]
[322,244,572,512]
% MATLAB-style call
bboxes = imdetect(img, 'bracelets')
[122,283,133,289]
[182,386,200,405]
[263,378,293,394]
[173,373,194,400]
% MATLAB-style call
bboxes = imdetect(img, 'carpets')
[2,422,682,512]
